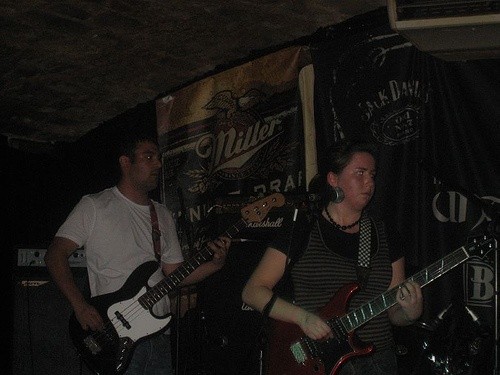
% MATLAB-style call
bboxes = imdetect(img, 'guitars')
[265,233,495,375]
[68,192,286,375]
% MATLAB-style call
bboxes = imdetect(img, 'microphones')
[309,188,344,203]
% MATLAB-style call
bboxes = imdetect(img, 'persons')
[44,135,232,375]
[240,138,423,375]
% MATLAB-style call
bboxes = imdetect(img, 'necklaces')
[322,202,365,231]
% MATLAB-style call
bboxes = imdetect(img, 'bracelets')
[261,292,279,318]
